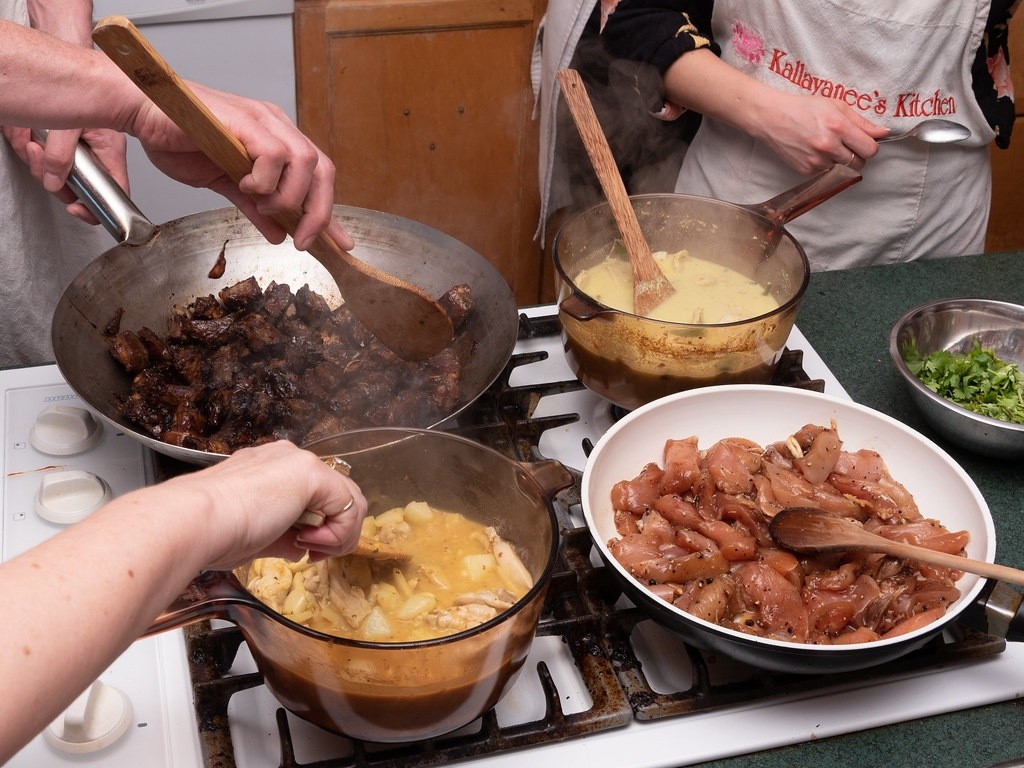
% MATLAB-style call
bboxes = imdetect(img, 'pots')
[30,126,518,465]
[553,163,862,413]
[580,383,1024,675]
[138,425,573,745]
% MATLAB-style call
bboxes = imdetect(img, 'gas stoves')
[166,303,1023,768]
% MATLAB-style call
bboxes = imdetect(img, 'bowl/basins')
[891,298,1024,448]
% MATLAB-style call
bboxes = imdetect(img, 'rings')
[845,153,855,166]
[332,495,354,517]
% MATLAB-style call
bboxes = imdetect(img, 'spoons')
[879,119,971,145]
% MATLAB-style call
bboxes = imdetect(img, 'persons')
[0,440,368,766]
[0,0,357,368]
[599,0,1018,274]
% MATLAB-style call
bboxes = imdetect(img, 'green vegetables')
[902,337,1024,425]
